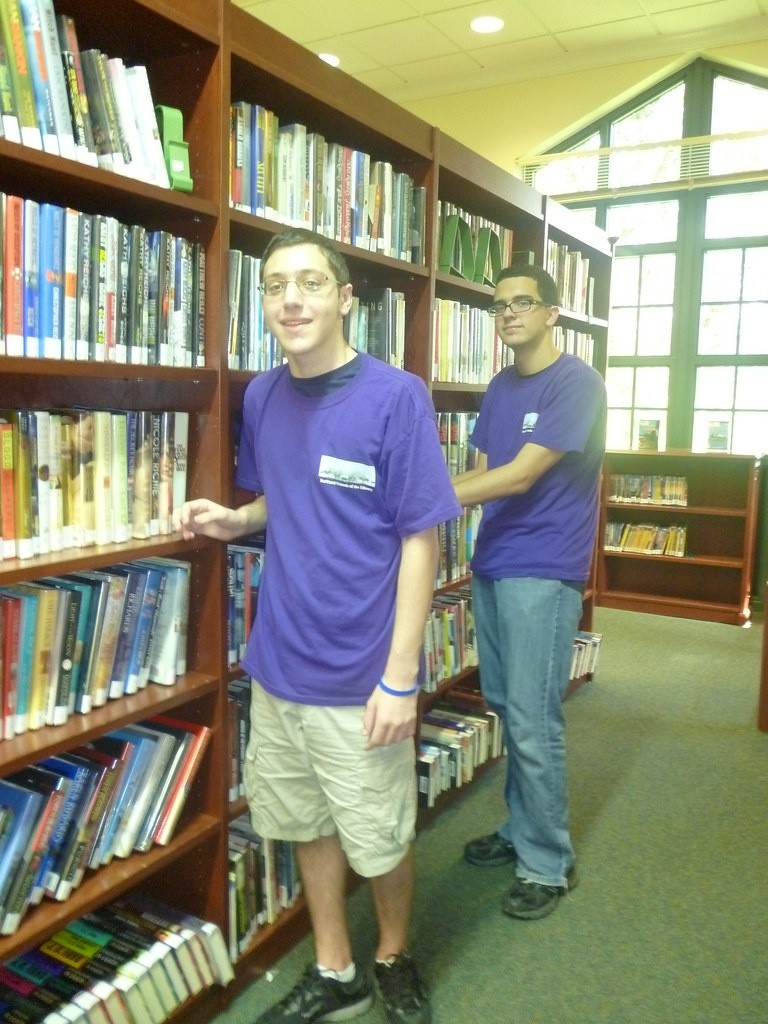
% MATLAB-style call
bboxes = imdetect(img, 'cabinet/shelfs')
[0,0,622,1024]
[592,451,762,627]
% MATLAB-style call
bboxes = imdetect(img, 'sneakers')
[370,952,432,1024]
[464,831,516,866]
[253,956,373,1024]
[500,865,577,918]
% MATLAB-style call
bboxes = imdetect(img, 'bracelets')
[377,675,418,696]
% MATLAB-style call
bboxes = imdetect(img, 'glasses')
[256,275,341,297]
[486,299,548,317]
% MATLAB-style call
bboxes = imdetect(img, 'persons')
[451,261,610,919]
[171,227,469,1024]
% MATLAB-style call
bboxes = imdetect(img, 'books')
[549,325,593,366]
[603,520,689,557]
[228,99,427,265]
[546,240,598,317]
[340,279,408,373]
[568,630,605,680]
[413,580,505,808]
[431,292,516,385]
[224,534,305,966]
[0,0,168,189]
[606,472,688,507]
[435,505,484,587]
[430,408,480,475]
[0,193,235,1024]
[228,248,288,373]
[438,201,515,287]
